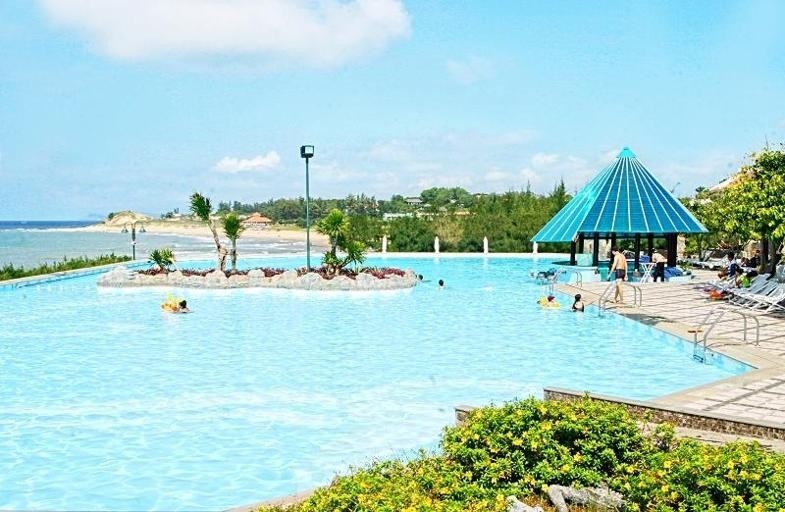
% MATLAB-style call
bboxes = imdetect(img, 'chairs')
[707,273,785,315]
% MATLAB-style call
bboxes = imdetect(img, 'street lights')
[300,144,314,272]
[121,220,146,260]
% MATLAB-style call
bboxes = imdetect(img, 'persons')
[439,280,445,289]
[537,272,546,280]
[607,246,628,304]
[572,294,585,312]
[651,247,665,282]
[419,274,423,281]
[536,296,556,305]
[179,300,190,312]
[722,250,760,289]
